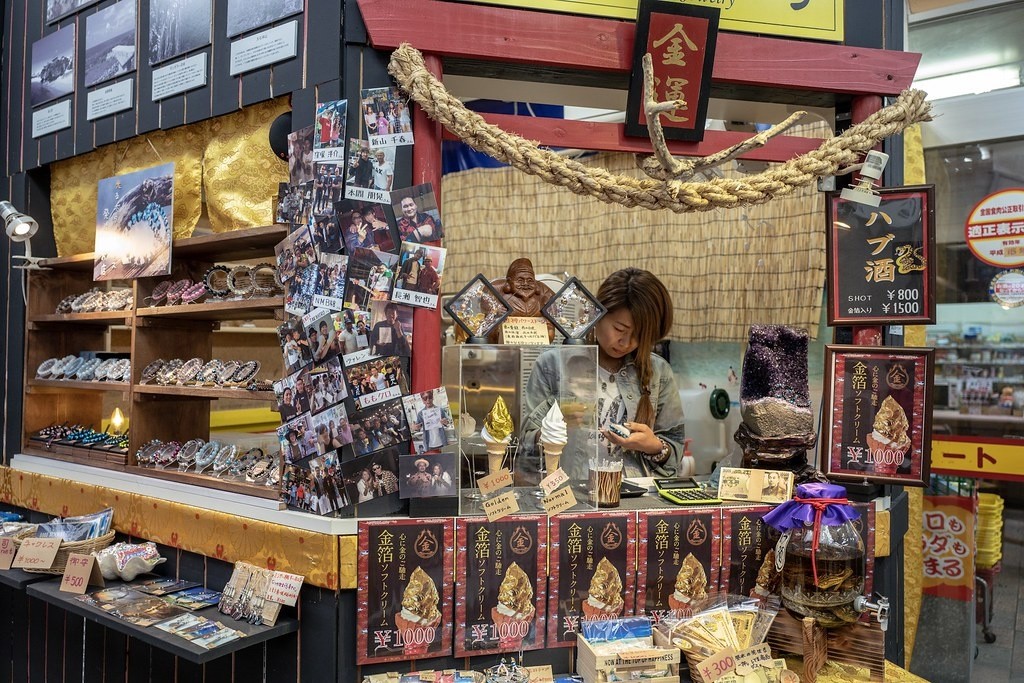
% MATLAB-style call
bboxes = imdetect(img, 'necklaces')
[81,577,217,618]
[599,361,622,382]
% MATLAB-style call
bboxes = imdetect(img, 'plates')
[571,478,647,498]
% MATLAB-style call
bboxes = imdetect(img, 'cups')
[590,467,621,507]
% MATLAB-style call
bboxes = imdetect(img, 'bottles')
[924,326,1024,407]
[779,483,867,608]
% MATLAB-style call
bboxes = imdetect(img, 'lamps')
[0,201,38,257]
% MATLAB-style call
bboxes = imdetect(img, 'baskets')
[13,524,116,574]
[213,272,284,298]
[974,492,1004,619]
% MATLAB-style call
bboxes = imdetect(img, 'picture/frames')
[821,345,934,488]
[827,185,940,329]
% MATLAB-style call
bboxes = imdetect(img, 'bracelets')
[39,426,129,448]
[36,354,131,380]
[641,438,668,460]
[143,358,273,391]
[136,439,279,486]
[152,264,285,301]
[57,289,133,311]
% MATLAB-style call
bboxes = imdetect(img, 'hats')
[361,147,370,152]
[344,319,352,323]
[309,327,317,338]
[415,458,429,467]
[285,428,298,441]
[319,321,327,331]
[369,461,384,468]
[423,257,432,262]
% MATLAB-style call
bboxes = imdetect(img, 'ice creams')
[491,561,535,646]
[750,549,783,610]
[481,395,514,475]
[668,552,708,616]
[866,395,912,473]
[540,398,567,476]
[395,565,443,655]
[583,556,625,620]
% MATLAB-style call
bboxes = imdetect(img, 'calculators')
[652,476,722,504]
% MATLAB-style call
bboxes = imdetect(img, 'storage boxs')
[578,628,681,682]
[442,344,599,513]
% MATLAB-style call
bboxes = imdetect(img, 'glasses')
[422,395,433,401]
[351,215,362,220]
[372,465,382,471]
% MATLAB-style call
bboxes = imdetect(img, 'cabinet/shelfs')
[24,576,295,682]
[21,223,290,501]
[931,340,1022,389]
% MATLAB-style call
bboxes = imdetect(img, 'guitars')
[349,277,388,302]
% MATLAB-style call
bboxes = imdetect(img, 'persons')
[516,268,685,481]
[482,260,557,344]
[763,472,785,496]
[273,91,452,512]
